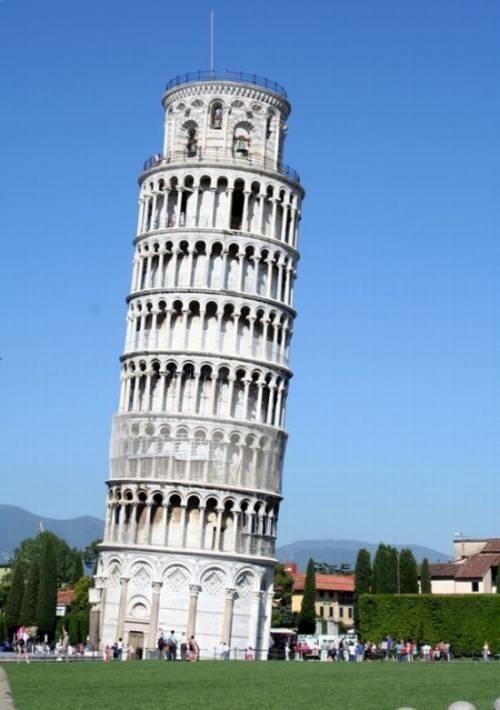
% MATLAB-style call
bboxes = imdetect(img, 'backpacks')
[158,638,166,650]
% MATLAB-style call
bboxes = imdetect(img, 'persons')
[481,640,490,663]
[287,632,452,663]
[221,642,230,661]
[157,630,200,663]
[245,647,253,661]
[0,622,142,664]
